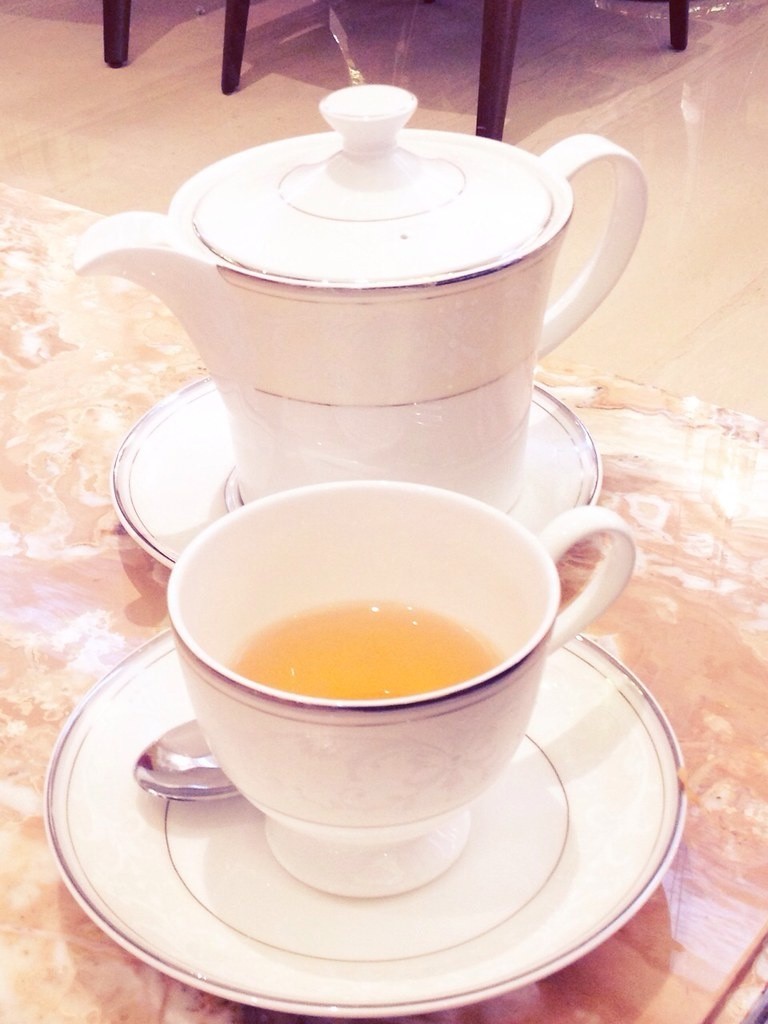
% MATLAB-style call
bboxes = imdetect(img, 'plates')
[111,373,601,571]
[46,634,685,1016]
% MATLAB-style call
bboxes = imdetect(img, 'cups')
[166,479,637,897]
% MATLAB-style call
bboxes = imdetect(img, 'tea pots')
[72,83,648,512]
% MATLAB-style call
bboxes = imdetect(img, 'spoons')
[134,719,240,800]
